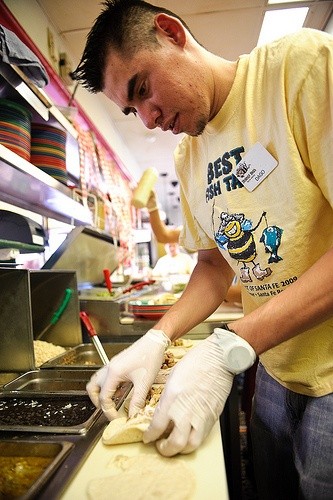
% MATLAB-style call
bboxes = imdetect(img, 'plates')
[1,97,70,186]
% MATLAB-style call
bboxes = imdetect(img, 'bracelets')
[148,206,159,214]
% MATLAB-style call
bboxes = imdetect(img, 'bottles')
[130,168,157,210]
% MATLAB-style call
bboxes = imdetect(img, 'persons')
[131,180,182,244]
[149,241,195,276]
[66,0,333,500]
[221,280,259,461]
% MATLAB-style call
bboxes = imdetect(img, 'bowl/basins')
[130,300,173,320]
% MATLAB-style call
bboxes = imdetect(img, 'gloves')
[86,329,171,423]
[143,330,236,457]
